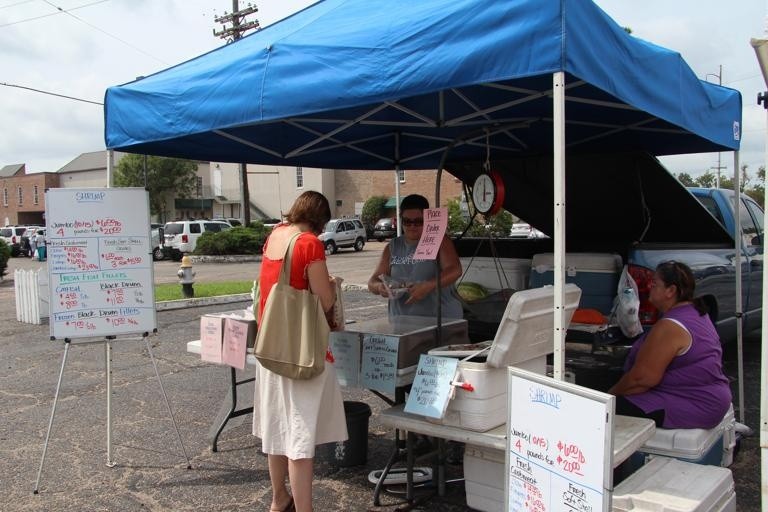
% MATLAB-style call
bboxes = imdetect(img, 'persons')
[250,191,350,512]
[607,260,731,431]
[364,191,470,457]
[33,229,47,262]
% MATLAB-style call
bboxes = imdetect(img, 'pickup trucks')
[566,188,764,373]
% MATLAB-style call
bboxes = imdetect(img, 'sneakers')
[400,438,432,460]
[444,442,460,466]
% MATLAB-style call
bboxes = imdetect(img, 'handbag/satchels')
[254,281,330,379]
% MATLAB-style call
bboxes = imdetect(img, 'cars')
[374,219,396,241]
[510,222,542,238]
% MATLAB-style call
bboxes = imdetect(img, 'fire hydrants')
[177,255,196,298]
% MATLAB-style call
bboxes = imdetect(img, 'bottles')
[621,287,640,337]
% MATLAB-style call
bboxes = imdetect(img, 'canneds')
[623,287,634,314]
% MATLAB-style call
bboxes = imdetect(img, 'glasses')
[399,215,424,227]
[666,260,687,278]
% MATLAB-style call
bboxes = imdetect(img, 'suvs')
[0,225,46,256]
[150,218,242,261]
[317,217,366,254]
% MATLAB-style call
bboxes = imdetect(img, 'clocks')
[468,168,510,219]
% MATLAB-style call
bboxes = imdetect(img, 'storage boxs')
[612,403,737,512]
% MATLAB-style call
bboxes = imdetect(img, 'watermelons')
[457,282,488,302]
